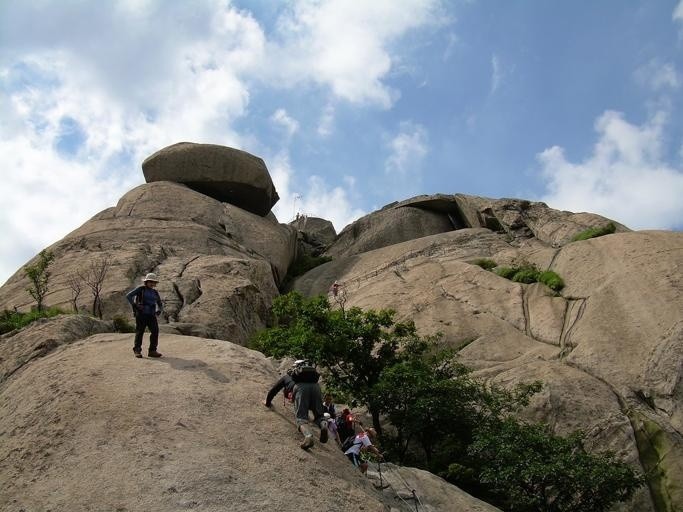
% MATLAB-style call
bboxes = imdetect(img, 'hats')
[366,427,377,439]
[141,273,160,283]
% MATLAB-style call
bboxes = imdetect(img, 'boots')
[320,420,328,443]
[300,423,314,449]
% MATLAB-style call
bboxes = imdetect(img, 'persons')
[331,280,343,300]
[344,427,385,475]
[299,214,303,218]
[284,390,292,400]
[258,358,328,450]
[323,412,343,449]
[336,407,355,454]
[322,392,341,427]
[123,272,164,360]
[295,213,299,221]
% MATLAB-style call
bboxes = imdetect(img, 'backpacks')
[288,365,320,383]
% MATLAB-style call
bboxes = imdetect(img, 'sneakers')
[148,351,162,357]
[133,350,142,358]
